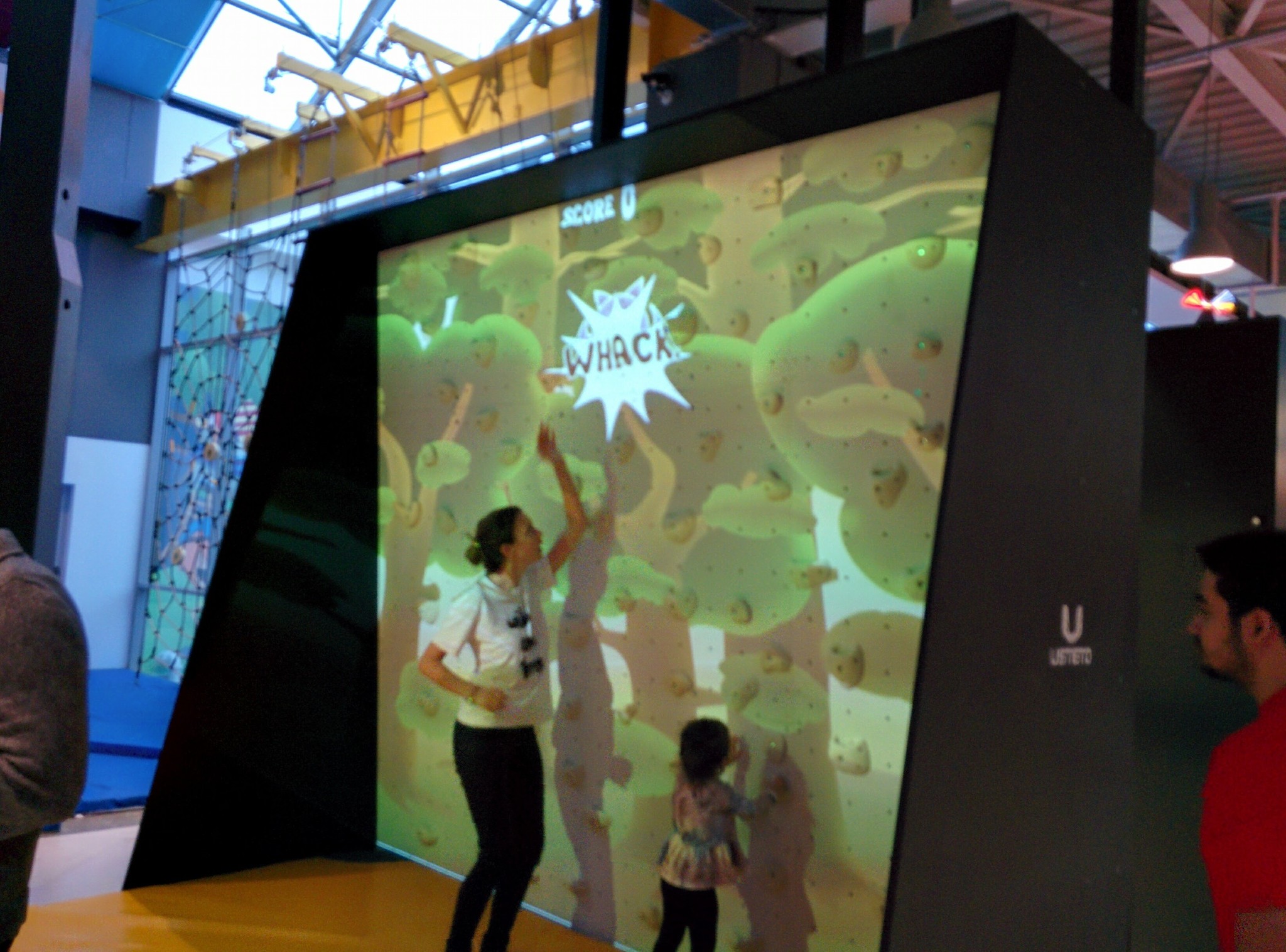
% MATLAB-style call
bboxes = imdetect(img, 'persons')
[646,717,792,952]
[0,531,92,951]
[419,422,588,952]
[1184,526,1285,952]
